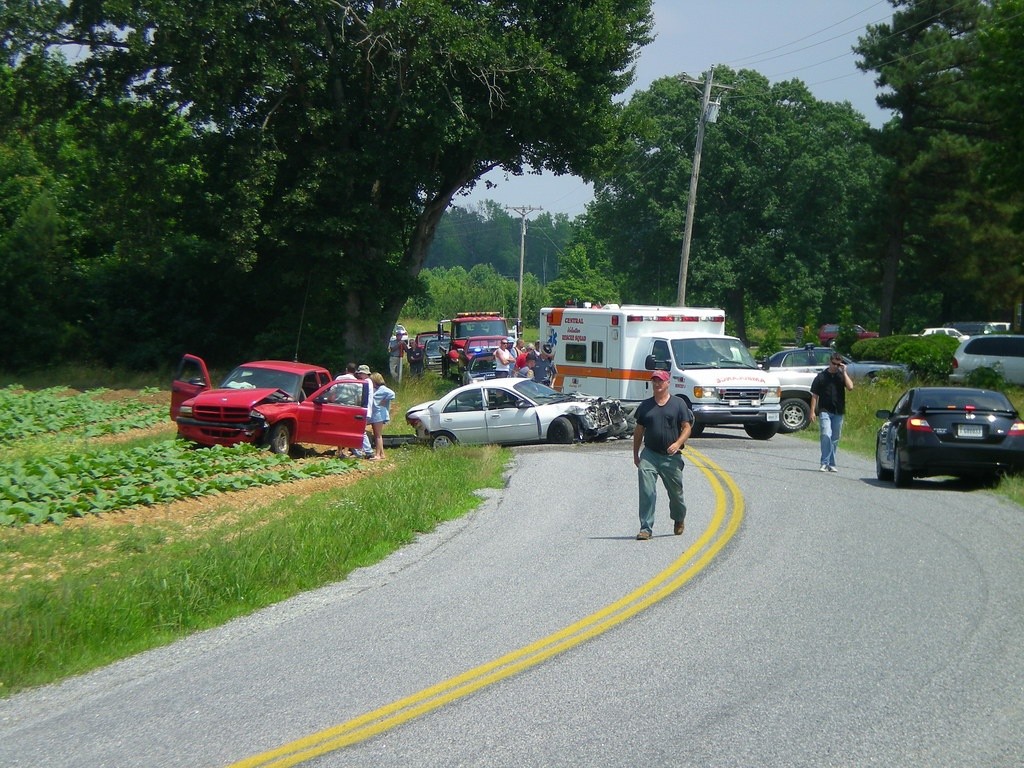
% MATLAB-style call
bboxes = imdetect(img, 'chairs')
[460,324,468,338]
[469,324,486,336]
[488,323,500,335]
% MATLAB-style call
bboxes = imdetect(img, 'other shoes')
[334,448,386,462]
[828,465,837,471]
[819,464,828,472]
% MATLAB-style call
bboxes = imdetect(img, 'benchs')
[446,402,477,411]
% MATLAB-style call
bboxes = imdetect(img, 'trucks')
[438,311,523,379]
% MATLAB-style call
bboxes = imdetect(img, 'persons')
[317,363,396,460]
[493,337,558,389]
[634,370,692,541]
[389,332,425,382]
[810,353,854,473]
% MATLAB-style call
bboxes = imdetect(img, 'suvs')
[819,323,879,347]
[763,343,914,433]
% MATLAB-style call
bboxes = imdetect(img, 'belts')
[391,355,399,357]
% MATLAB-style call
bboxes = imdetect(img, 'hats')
[535,340,540,346]
[650,368,669,381]
[543,344,552,354]
[355,364,371,375]
[396,330,403,335]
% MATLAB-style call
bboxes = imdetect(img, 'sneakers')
[637,532,653,540]
[674,520,684,534]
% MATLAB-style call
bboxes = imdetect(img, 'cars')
[919,327,970,343]
[415,331,451,372]
[457,335,516,387]
[875,386,1024,487]
[406,377,627,454]
[169,354,368,457]
[390,325,408,345]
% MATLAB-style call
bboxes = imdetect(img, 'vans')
[949,334,1024,384]
[943,321,1011,336]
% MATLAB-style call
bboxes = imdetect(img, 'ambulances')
[540,299,782,440]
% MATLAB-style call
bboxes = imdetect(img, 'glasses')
[652,380,664,385]
[830,360,840,366]
[502,343,508,344]
